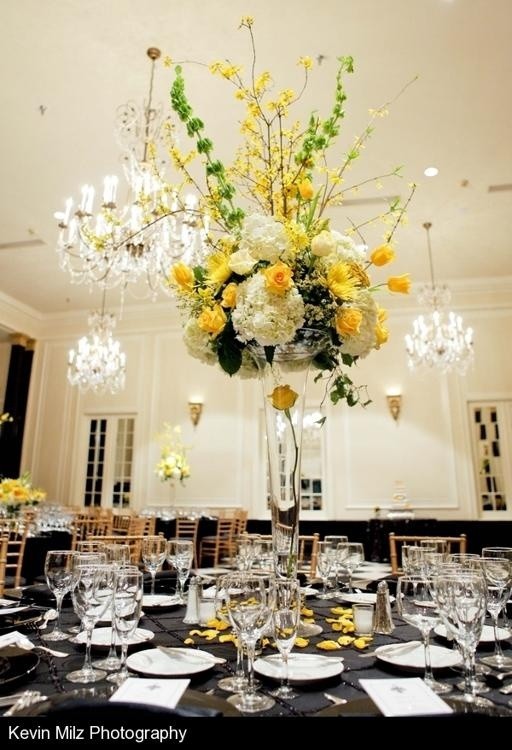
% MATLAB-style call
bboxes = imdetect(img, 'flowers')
[155,423,191,484]
[265,385,298,571]
[76,16,420,431]
[0,471,46,508]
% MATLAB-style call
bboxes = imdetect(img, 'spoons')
[38,607,58,630]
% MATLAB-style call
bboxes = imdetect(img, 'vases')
[249,350,325,581]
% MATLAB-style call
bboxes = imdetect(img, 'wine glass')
[107,543,132,566]
[106,571,143,683]
[79,542,106,554]
[231,532,273,574]
[317,542,333,600]
[470,560,512,667]
[480,547,512,629]
[336,543,365,597]
[416,547,435,587]
[325,534,348,591]
[65,565,106,684]
[170,539,195,603]
[397,575,455,694]
[401,545,414,583]
[269,580,301,699]
[142,539,166,597]
[226,577,277,713]
[70,554,107,635]
[40,551,81,641]
[451,551,480,563]
[440,576,494,706]
[216,578,266,693]
[420,540,449,554]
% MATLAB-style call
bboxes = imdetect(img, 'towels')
[0,630,35,651]
[0,607,28,614]
[358,676,454,717]
[108,678,191,710]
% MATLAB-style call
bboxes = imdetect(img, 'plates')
[130,645,217,677]
[0,607,42,632]
[254,652,347,681]
[1,645,40,685]
[77,627,155,648]
[337,587,396,608]
[141,594,178,608]
[373,641,463,671]
[431,615,510,647]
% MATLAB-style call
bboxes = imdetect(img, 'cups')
[352,604,373,636]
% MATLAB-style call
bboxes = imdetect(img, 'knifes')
[358,640,420,661]
[281,656,343,670]
[158,644,227,668]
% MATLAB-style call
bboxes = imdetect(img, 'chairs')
[297,533,320,578]
[1,533,30,588]
[236,512,248,534]
[389,534,466,574]
[0,534,9,595]
[172,519,200,570]
[71,509,155,564]
[200,518,234,567]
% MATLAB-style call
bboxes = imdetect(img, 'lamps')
[65,278,127,399]
[404,223,480,377]
[188,401,202,426]
[54,46,210,304]
[386,395,402,420]
